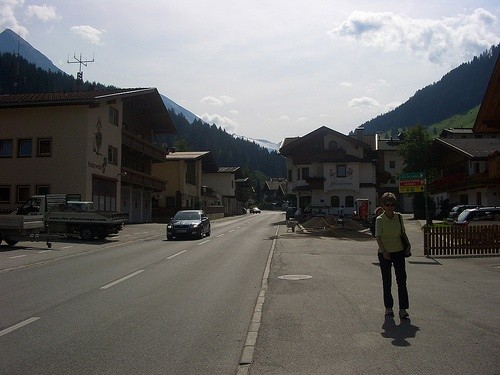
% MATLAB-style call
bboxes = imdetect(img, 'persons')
[369,207,384,238]
[375,192,409,317]
[294,208,301,220]
[337,205,345,229]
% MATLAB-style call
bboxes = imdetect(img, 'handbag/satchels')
[398,214,411,257]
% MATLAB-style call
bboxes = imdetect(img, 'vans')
[65,201,95,212]
[454,207,500,241]
[286,207,297,221]
[446,205,479,223]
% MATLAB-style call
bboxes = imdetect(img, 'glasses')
[383,202,396,207]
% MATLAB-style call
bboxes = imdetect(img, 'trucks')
[0,212,46,245]
[11,196,130,241]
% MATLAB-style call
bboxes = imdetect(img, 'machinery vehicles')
[350,198,376,227]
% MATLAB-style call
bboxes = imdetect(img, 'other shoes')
[399,309,409,317]
[386,308,393,315]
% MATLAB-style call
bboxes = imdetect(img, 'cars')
[166,210,210,240]
[249,207,262,214]
